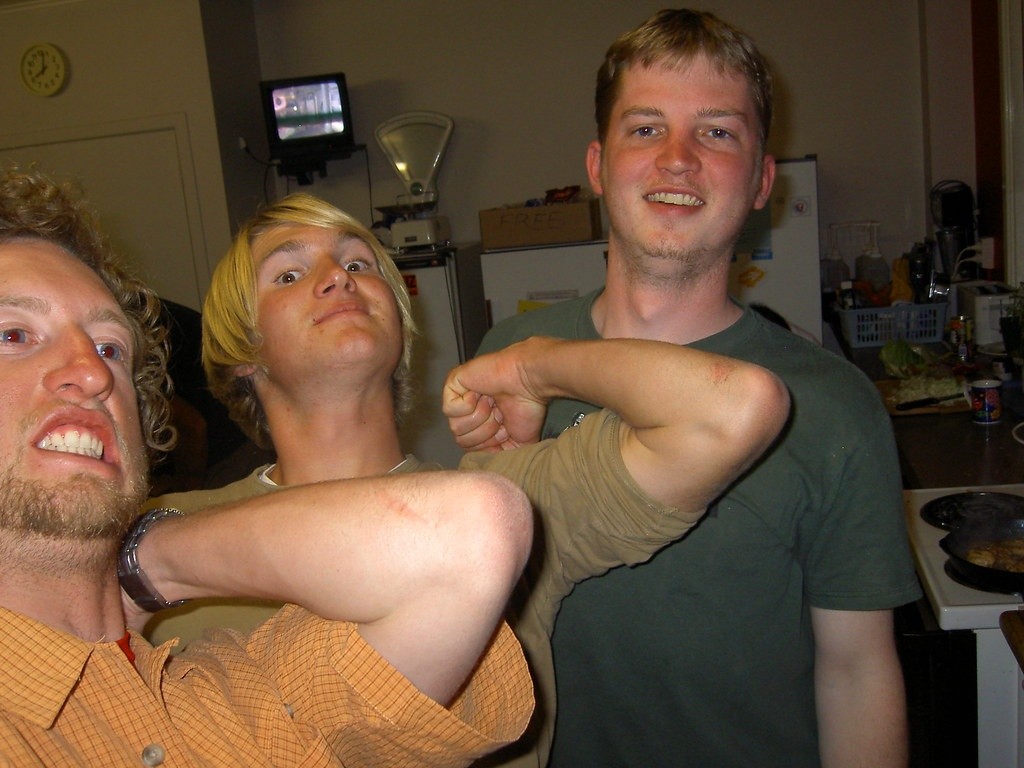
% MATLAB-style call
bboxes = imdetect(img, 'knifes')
[895,393,964,411]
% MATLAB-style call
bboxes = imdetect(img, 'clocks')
[17,41,68,98]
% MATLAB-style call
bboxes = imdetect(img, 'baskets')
[833,301,947,348]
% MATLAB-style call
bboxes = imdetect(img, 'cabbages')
[878,338,950,377]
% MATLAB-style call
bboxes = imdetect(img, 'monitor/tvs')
[260,72,356,160]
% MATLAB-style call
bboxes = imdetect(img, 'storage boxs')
[478,198,602,251]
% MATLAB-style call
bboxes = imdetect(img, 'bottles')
[841,281,856,310]
[955,314,968,361]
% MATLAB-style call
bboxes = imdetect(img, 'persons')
[135,193,791,767]
[0,169,536,768]
[469,9,924,768]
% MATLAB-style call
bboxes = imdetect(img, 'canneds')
[950,315,973,345]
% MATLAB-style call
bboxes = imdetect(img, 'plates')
[977,342,1008,358]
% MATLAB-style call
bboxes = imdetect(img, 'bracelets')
[116,508,190,611]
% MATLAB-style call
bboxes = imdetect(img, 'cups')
[970,379,1002,424]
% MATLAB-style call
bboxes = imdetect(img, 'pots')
[938,516,1024,590]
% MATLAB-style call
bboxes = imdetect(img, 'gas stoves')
[900,482,1024,631]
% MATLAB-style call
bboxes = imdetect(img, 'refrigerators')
[712,153,823,351]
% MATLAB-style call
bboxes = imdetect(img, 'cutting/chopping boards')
[871,379,970,416]
